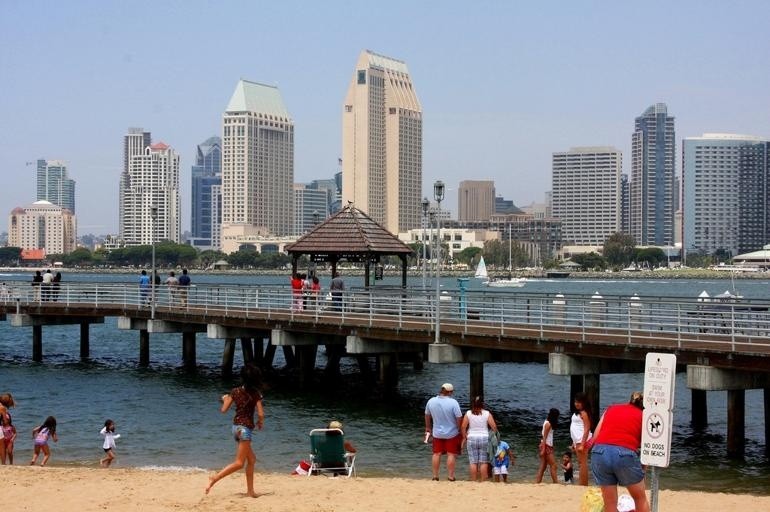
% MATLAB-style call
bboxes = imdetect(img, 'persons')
[0,394,17,465]
[535,408,560,484]
[491,435,515,482]
[461,393,496,482]
[30,416,58,467]
[569,393,593,486]
[41,270,54,301]
[582,392,651,512]
[290,271,345,313]
[325,421,356,477]
[99,419,120,468]
[32,271,43,301]
[139,267,190,310]
[560,452,574,484]
[205,363,264,499]
[52,272,62,301]
[424,383,466,480]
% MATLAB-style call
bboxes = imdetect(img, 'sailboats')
[483,221,528,288]
[474,256,489,279]
[714,250,744,304]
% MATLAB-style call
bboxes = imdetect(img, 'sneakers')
[431,477,439,481]
[448,477,455,482]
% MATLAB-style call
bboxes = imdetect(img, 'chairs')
[307,428,356,478]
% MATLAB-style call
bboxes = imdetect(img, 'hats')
[441,382,456,392]
[329,421,343,428]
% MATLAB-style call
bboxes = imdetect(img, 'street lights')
[149,206,159,320]
[312,209,321,228]
[433,178,446,344]
[421,196,429,316]
